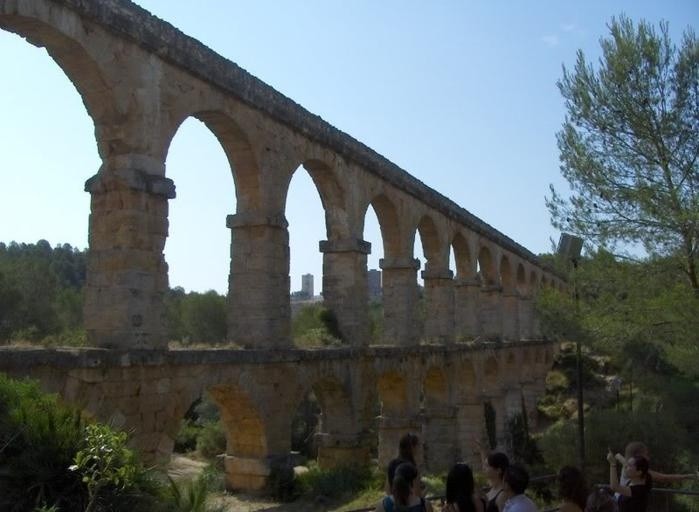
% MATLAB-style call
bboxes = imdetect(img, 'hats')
[395,462,421,482]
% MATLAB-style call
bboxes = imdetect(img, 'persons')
[557,441,698,511]
[376,435,535,510]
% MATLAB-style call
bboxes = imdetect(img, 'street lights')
[554,232,587,473]
